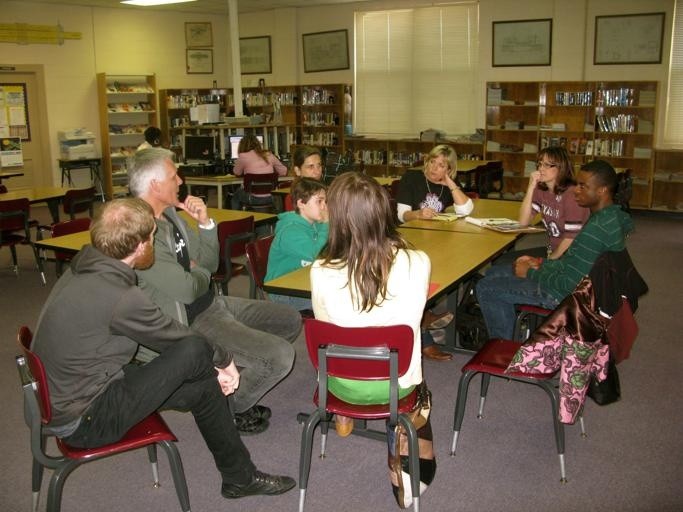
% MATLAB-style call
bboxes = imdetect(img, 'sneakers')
[336,419,354,437]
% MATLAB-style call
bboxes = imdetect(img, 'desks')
[32,207,279,300]
[261,198,541,442]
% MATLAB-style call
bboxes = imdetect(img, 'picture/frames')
[186,49,213,75]
[301,30,349,73]
[0,83,31,143]
[238,36,273,75]
[593,12,664,66]
[184,21,213,48]
[492,19,553,68]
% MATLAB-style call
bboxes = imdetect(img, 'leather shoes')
[233,405,271,435]
[423,343,453,361]
[421,309,454,330]
[222,471,296,499]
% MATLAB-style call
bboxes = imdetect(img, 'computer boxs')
[180,164,226,177]
[228,164,235,175]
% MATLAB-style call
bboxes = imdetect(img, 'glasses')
[537,160,556,168]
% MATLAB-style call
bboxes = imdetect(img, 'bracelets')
[447,186,461,191]
[536,256,545,265]
[413,210,419,220]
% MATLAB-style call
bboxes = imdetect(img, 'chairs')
[297,318,419,512]
[459,161,505,196]
[235,173,280,211]
[0,172,98,286]
[209,216,255,296]
[17,327,191,511]
[245,232,273,299]
[449,254,629,484]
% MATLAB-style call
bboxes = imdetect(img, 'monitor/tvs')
[229,135,264,160]
[183,135,216,166]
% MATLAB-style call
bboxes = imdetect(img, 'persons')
[473,159,633,341]
[230,134,285,209]
[129,148,299,433]
[309,171,432,439]
[284,145,324,212]
[419,309,457,363]
[389,145,472,224]
[32,198,296,497]
[264,178,332,310]
[487,145,590,267]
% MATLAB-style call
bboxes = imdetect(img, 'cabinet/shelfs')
[159,84,298,153]
[483,81,536,199]
[299,84,345,148]
[650,149,682,213]
[289,130,486,190]
[537,81,594,168]
[592,81,659,211]
[97,72,158,201]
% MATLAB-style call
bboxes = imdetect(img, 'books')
[637,165,682,183]
[346,128,483,184]
[106,80,154,193]
[166,89,338,158]
[486,87,637,200]
[462,214,546,235]
[428,212,458,222]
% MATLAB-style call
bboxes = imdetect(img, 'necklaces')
[426,177,442,201]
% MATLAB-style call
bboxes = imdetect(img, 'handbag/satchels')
[606,300,638,365]
[456,273,489,351]
[586,332,620,405]
[385,381,435,508]
